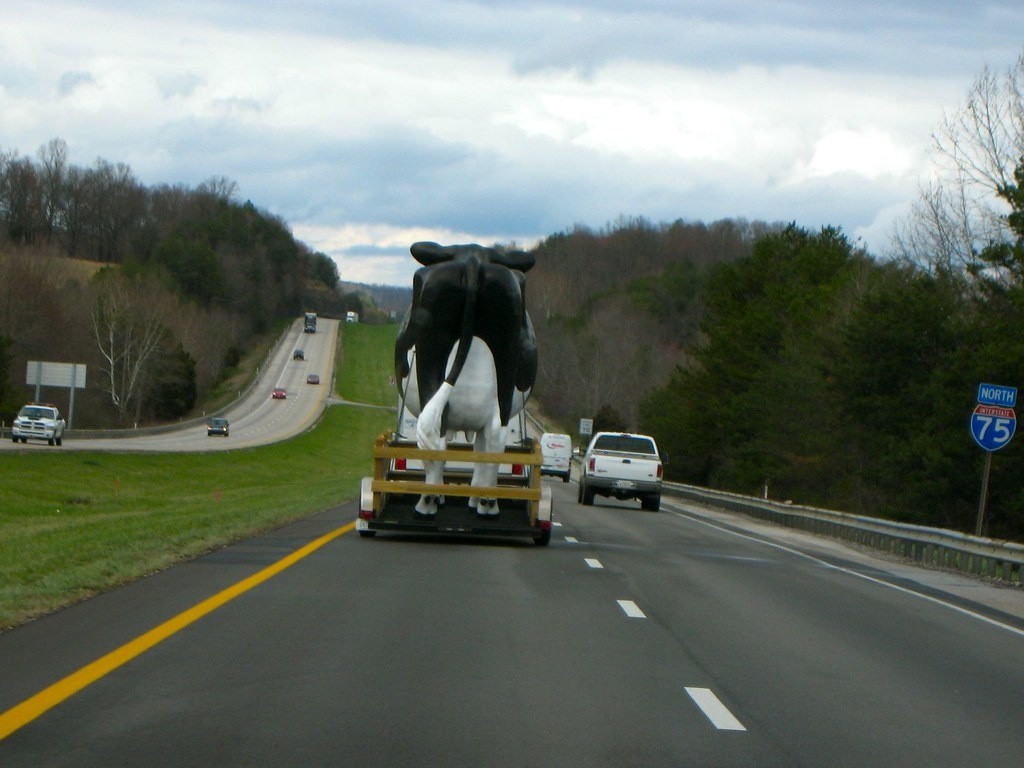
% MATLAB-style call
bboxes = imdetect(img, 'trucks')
[391,395,532,491]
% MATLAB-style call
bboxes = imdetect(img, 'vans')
[539,432,573,482]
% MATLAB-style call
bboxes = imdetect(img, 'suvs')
[293,349,304,360]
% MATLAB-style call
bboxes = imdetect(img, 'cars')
[206,418,230,436]
[273,388,286,399]
[12,402,66,446]
[307,375,319,384]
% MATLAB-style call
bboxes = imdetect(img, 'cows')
[394,241,539,521]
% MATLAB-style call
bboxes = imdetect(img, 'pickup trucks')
[578,431,672,511]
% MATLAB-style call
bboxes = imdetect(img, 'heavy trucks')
[347,311,359,322]
[305,312,317,333]
